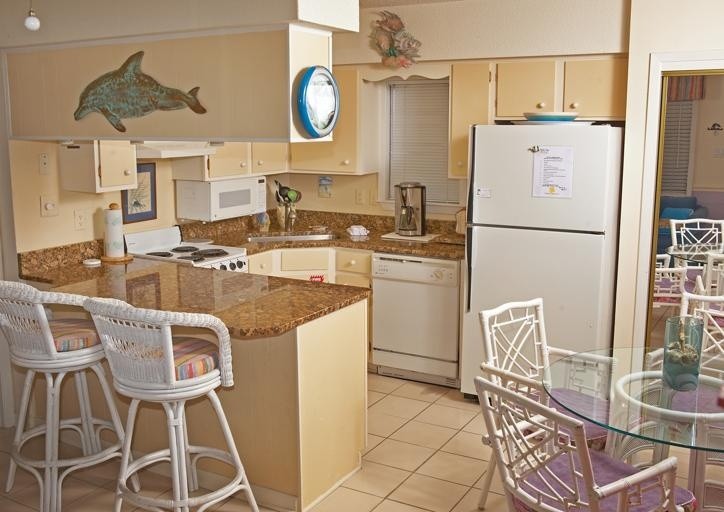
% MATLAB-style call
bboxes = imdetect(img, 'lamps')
[23,3,45,32]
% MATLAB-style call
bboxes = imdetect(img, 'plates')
[524,109,578,120]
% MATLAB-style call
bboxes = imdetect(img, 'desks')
[662,243,724,273]
[541,345,722,506]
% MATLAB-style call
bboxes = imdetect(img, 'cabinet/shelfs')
[292,68,361,174]
[495,57,629,122]
[201,144,290,180]
[448,61,490,178]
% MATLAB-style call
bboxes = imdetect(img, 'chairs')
[0,279,141,507]
[668,216,723,274]
[652,253,683,308]
[479,295,620,507]
[683,275,724,310]
[474,376,679,506]
[645,308,724,376]
[84,294,260,507]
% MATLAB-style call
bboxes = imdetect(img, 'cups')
[663,316,705,393]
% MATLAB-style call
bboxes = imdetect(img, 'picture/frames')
[120,161,157,227]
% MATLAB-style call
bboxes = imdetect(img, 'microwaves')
[177,175,266,222]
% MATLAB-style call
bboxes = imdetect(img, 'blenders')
[393,181,426,236]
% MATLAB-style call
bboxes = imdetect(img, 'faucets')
[279,202,298,232]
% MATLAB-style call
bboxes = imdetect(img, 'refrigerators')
[460,125,621,450]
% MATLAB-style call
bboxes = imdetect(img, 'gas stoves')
[141,245,247,266]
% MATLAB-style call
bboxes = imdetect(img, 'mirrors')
[643,71,724,441]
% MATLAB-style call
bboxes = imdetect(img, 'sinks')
[247,233,339,243]
